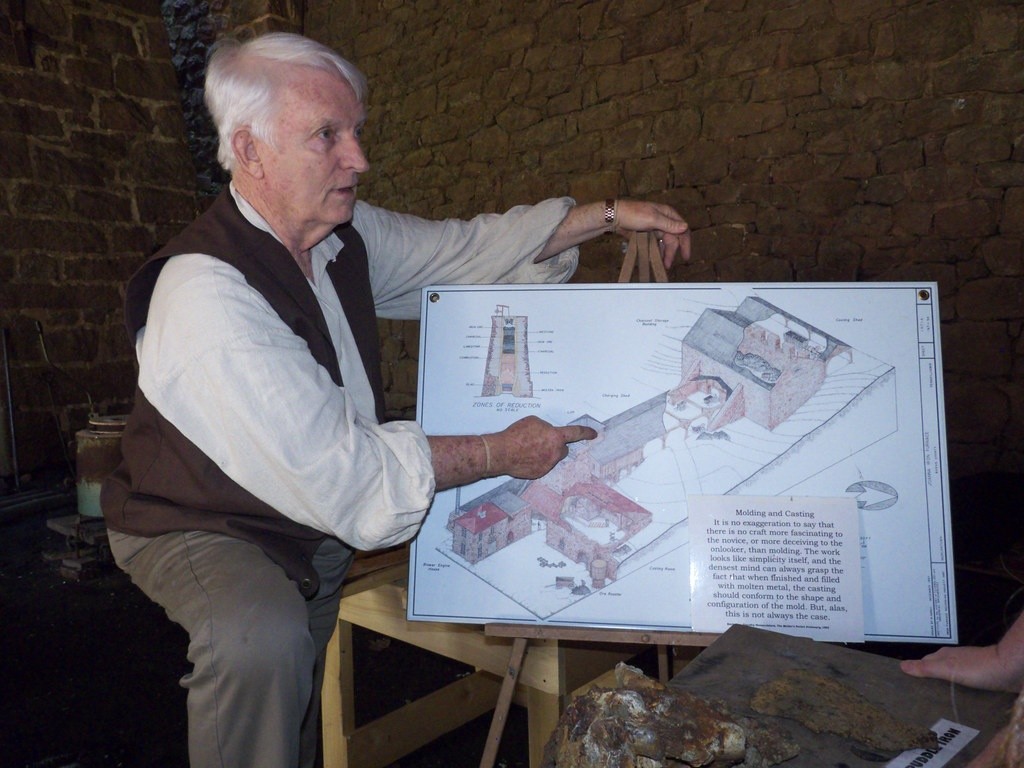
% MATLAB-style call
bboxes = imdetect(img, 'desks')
[315,546,704,767]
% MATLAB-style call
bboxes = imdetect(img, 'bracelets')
[478,435,490,479]
[603,197,615,235]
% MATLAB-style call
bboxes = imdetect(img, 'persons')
[901,605,1024,697]
[98,32,692,768]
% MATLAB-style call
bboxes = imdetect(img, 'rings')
[658,233,669,242]
